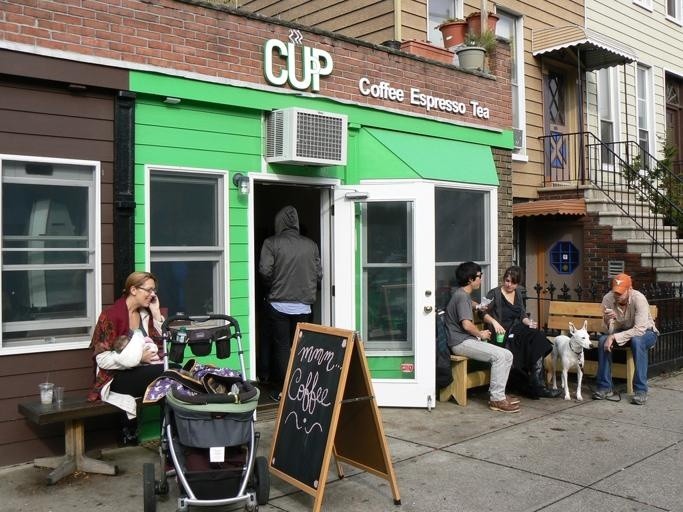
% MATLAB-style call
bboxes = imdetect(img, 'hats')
[613,274,632,295]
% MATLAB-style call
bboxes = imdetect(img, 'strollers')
[143,315,271,512]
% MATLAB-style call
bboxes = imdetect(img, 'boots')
[530,355,562,400]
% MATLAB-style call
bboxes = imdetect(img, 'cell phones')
[605,309,613,314]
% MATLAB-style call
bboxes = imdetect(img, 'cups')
[496,333,505,343]
[38,382,54,405]
[53,386,65,404]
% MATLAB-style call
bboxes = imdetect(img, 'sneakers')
[267,391,282,402]
[489,400,520,413]
[633,392,648,405]
[592,389,613,399]
[256,375,266,389]
[488,395,520,405]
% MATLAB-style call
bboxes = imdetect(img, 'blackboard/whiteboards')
[271,329,348,491]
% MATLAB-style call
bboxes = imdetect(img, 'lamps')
[233,173,249,196]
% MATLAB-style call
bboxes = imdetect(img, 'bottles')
[176,327,188,343]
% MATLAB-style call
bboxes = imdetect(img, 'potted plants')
[435,11,499,72]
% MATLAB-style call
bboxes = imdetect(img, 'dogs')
[551,320,594,401]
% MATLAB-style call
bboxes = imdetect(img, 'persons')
[258,205,323,401]
[113,335,160,367]
[592,273,661,405]
[89,271,186,476]
[478,266,563,400]
[444,262,520,412]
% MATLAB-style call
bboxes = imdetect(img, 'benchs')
[544,301,658,395]
[18,388,167,485]
[439,323,492,405]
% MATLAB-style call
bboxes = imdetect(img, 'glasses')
[135,286,158,294]
[475,275,481,279]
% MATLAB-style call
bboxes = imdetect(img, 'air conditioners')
[265,106,348,165]
